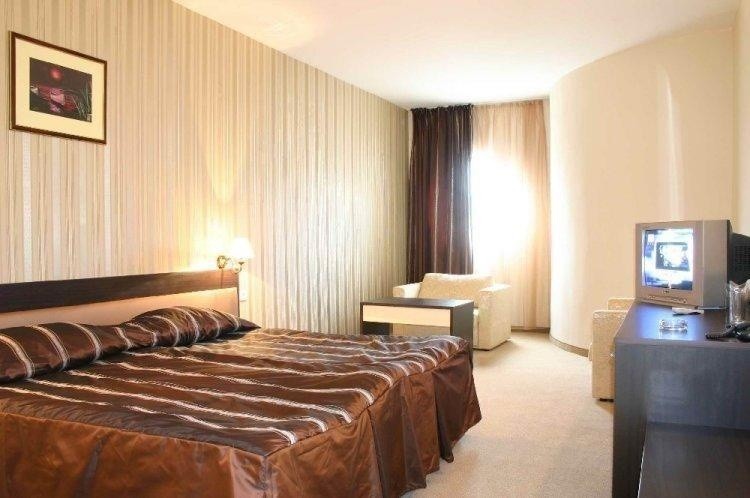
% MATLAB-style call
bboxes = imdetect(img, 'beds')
[0,269,482,498]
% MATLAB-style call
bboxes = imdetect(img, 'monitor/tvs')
[635,219,750,309]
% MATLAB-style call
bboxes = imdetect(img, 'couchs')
[392,272,514,350]
[588,298,635,402]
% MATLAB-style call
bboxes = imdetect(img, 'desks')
[359,297,475,371]
[611,301,750,497]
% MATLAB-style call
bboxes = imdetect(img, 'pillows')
[122,305,261,351]
[0,322,134,385]
[417,273,491,308]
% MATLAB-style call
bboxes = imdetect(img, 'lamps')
[217,238,255,275]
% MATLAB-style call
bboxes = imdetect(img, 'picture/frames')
[8,30,108,145]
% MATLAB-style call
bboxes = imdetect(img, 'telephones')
[706,321,750,345]
